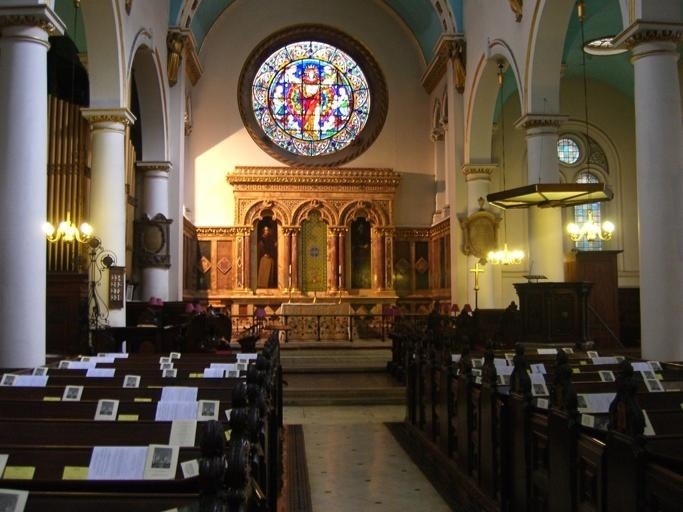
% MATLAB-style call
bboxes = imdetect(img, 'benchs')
[404,349,682,512]
[0,351,286,512]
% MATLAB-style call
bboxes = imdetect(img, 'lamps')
[40,1,96,244]
[565,1,617,245]
